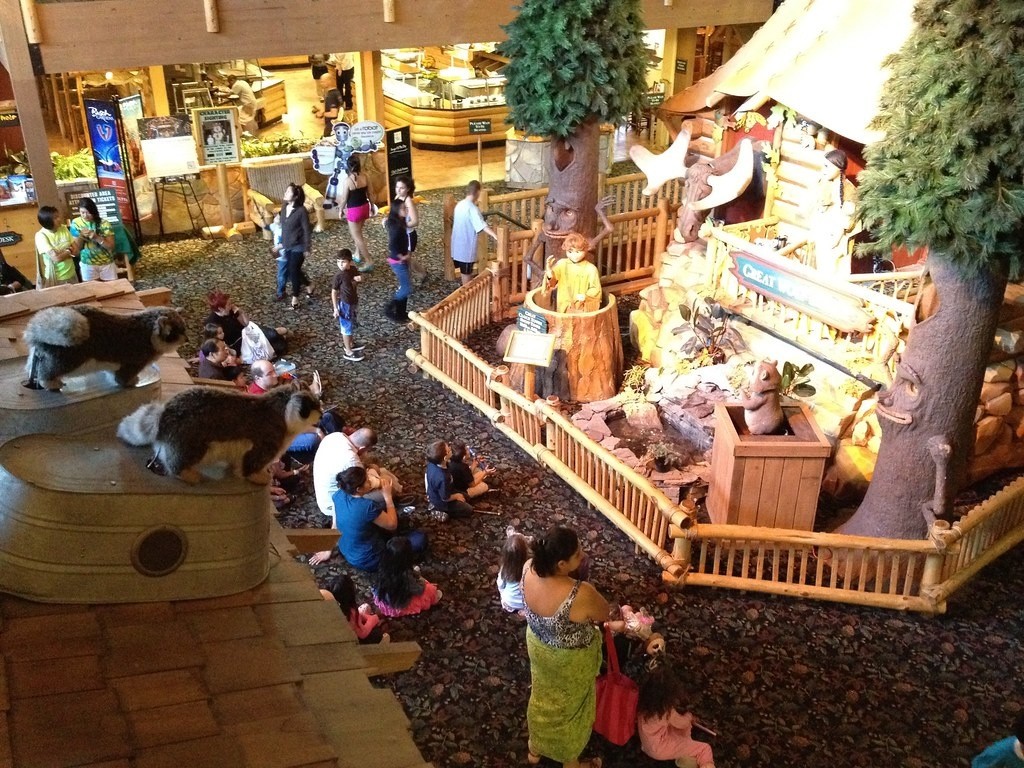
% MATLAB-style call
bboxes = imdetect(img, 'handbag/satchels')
[590,625,638,746]
[238,320,275,365]
[34,234,55,290]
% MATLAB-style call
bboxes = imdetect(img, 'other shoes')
[581,758,601,767]
[385,298,409,323]
[427,502,448,522]
[351,255,361,263]
[527,751,540,763]
[307,369,324,397]
[357,260,376,273]
[343,343,365,351]
[343,352,365,362]
[305,282,316,300]
[290,295,303,312]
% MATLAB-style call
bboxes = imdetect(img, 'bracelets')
[293,470,299,475]
[66,248,75,257]
[316,428,321,433]
[99,237,104,245]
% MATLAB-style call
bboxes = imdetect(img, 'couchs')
[241,156,326,241]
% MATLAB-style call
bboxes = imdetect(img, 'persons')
[541,234,602,314]
[637,670,715,768]
[70,197,117,282]
[35,207,79,286]
[812,150,863,276]
[307,52,354,137]
[213,75,256,132]
[497,534,531,621]
[568,554,590,581]
[339,156,374,272]
[521,529,610,768]
[331,249,366,362]
[188,290,496,641]
[254,184,316,310]
[382,177,429,323]
[451,180,497,286]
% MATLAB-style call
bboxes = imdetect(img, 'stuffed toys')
[610,605,665,657]
